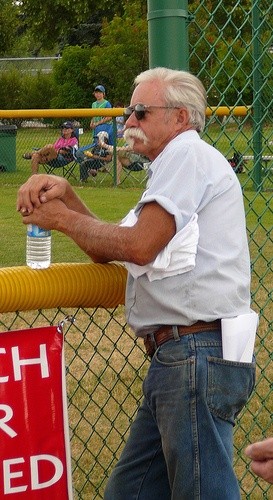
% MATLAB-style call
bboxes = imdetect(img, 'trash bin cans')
[0,124,18,173]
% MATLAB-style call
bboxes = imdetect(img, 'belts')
[143,319,221,355]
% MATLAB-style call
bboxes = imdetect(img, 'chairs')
[34,124,151,185]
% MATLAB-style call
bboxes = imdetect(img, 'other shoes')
[22,153,33,159]
[80,178,87,183]
[88,169,97,176]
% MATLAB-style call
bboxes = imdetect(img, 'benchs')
[232,142,273,170]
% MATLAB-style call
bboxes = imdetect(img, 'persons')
[114,101,125,138]
[80,131,151,186]
[89,85,114,146]
[22,121,79,174]
[228,149,245,176]
[244,437,273,485]
[17,66,260,500]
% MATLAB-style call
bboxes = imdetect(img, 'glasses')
[62,127,72,129]
[124,103,182,120]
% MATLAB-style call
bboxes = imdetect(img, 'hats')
[59,121,74,128]
[93,131,109,140]
[94,85,105,93]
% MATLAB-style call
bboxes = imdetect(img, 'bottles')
[25,223,51,270]
